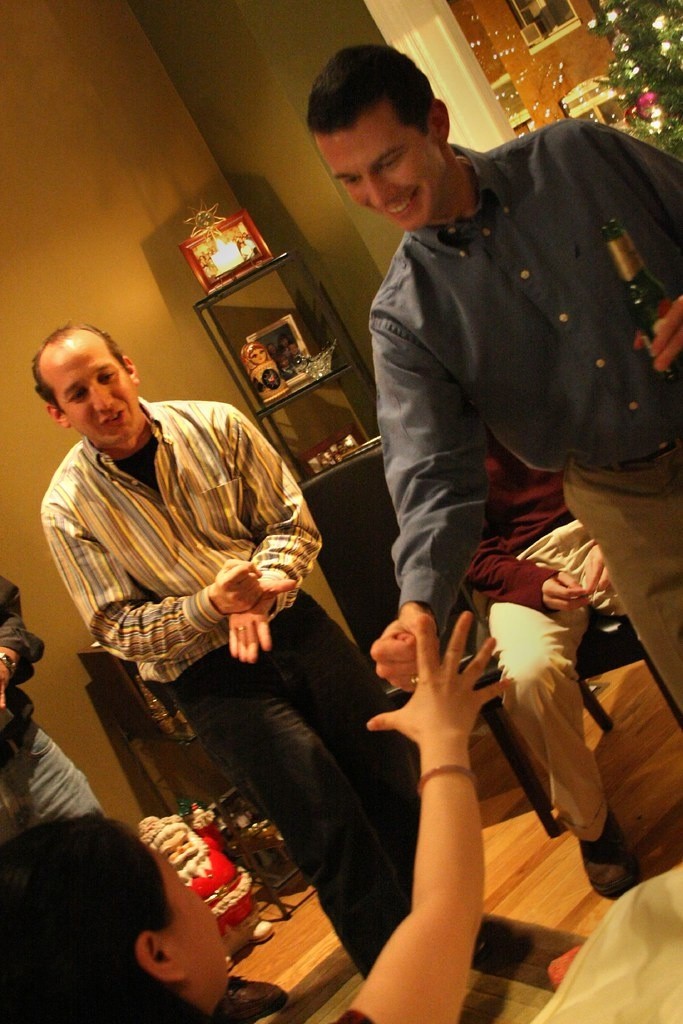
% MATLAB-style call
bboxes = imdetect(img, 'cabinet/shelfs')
[191,251,408,508]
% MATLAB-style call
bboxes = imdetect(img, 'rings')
[237,626,246,632]
[256,594,259,601]
[411,675,418,687]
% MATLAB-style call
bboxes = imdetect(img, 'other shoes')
[471,916,495,965]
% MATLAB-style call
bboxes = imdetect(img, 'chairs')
[440,574,683,837]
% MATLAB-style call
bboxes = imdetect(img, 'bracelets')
[0,653,16,678]
[416,763,478,795]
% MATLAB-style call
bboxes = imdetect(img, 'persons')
[29,319,491,982]
[241,341,289,406]
[266,336,301,379]
[0,575,104,843]
[462,422,640,900]
[137,802,276,973]
[199,233,260,281]
[0,611,514,1024]
[308,45,683,712]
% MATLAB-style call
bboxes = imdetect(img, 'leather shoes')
[579,809,640,897]
[214,975,287,1023]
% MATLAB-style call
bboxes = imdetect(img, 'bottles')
[599,214,683,381]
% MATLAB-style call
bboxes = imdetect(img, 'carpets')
[255,914,588,1024]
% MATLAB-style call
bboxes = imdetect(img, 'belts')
[618,438,683,463]
